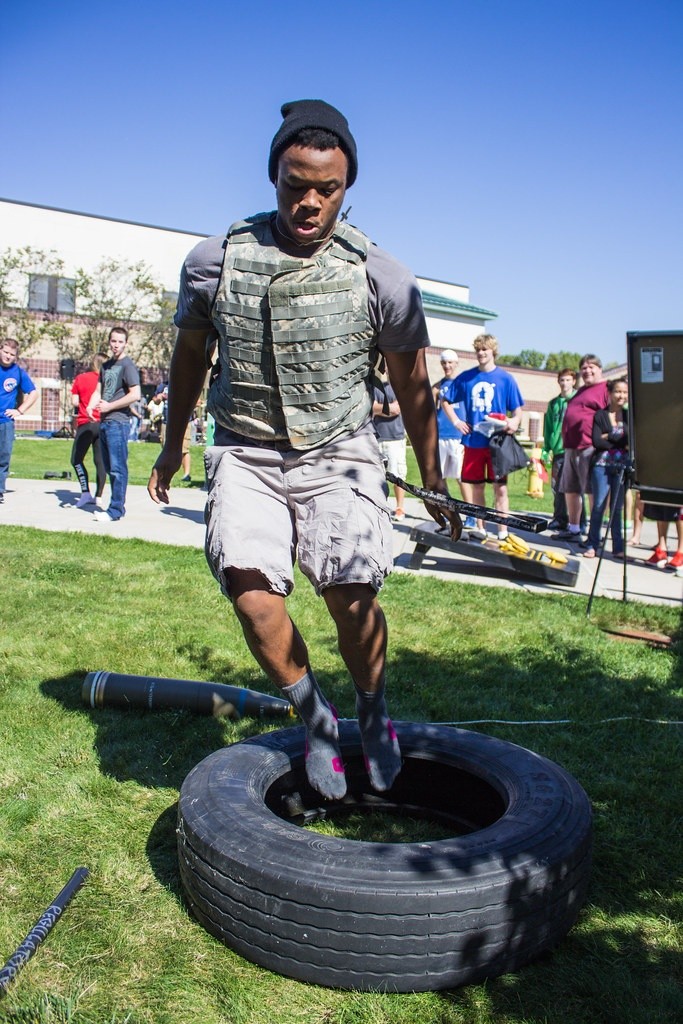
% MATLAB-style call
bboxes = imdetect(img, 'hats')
[268,99,358,189]
[440,350,458,361]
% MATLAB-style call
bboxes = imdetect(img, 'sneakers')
[551,529,581,542]
[579,538,603,548]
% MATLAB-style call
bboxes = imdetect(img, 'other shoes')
[393,508,405,521]
[182,474,191,482]
[547,519,569,530]
[463,516,476,528]
[666,552,683,570]
[497,530,508,540]
[645,549,668,566]
[0,493,4,504]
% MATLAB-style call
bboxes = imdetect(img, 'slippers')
[583,548,597,558]
[626,539,645,547]
[613,552,635,562]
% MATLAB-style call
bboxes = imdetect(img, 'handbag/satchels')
[489,432,530,481]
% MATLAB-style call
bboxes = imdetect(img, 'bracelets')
[17,409,22,414]
[453,419,460,426]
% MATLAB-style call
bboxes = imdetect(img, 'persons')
[148,99,463,801]
[129,383,168,443]
[0,339,38,504]
[373,372,409,520]
[542,368,586,535]
[588,488,683,570]
[70,353,109,506]
[180,398,200,481]
[86,327,142,523]
[550,355,611,548]
[439,335,524,541]
[584,379,634,562]
[430,350,477,528]
